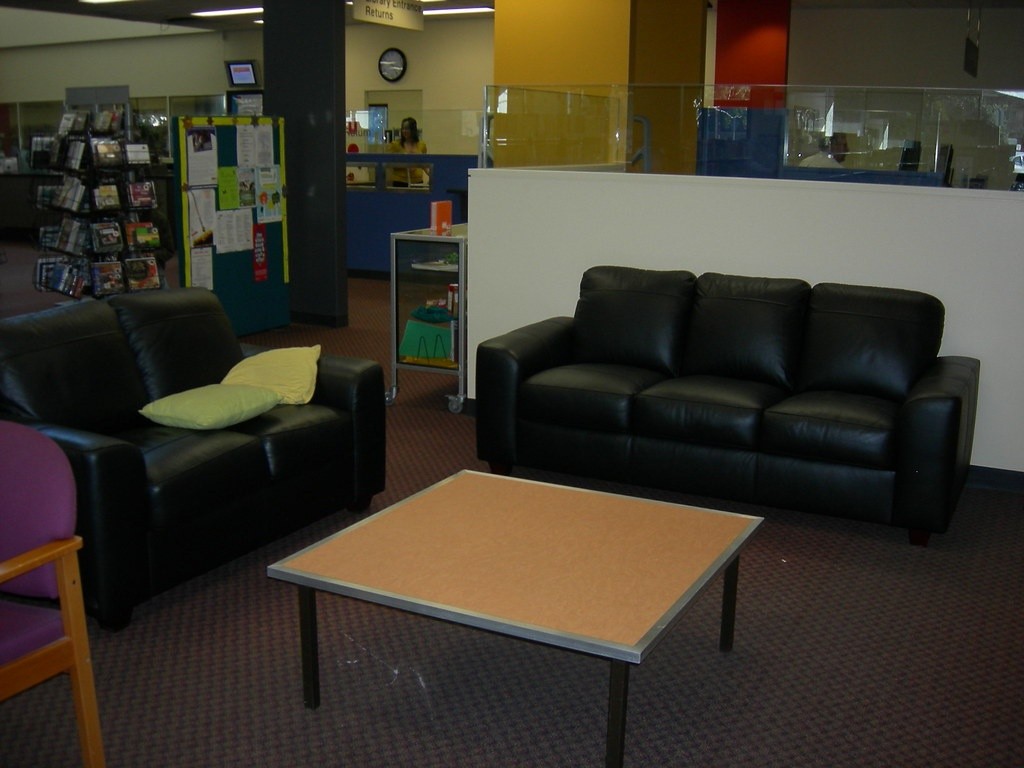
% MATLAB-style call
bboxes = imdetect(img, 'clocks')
[378,46,407,82]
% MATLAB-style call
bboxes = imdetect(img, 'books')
[126,253,161,289]
[92,256,123,295]
[90,222,124,253]
[90,135,151,167]
[126,214,161,250]
[57,108,122,134]
[36,258,90,297]
[92,183,121,209]
[34,177,90,214]
[126,181,158,210]
[35,218,86,254]
[31,135,85,170]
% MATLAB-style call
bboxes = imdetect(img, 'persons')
[798,126,850,169]
[385,115,430,188]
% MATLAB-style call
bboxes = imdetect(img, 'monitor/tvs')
[224,59,262,88]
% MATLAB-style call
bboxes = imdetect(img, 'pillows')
[138,384,286,434]
[220,345,322,406]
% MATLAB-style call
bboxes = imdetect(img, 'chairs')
[0,422,107,767]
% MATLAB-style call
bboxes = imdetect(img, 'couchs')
[0,286,388,636]
[473,262,983,550]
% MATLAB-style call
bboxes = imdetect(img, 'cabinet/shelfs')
[30,125,165,301]
[389,222,468,413]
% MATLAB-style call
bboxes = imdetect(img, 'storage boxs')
[431,201,452,236]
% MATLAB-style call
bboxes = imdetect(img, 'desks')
[264,468,768,768]
